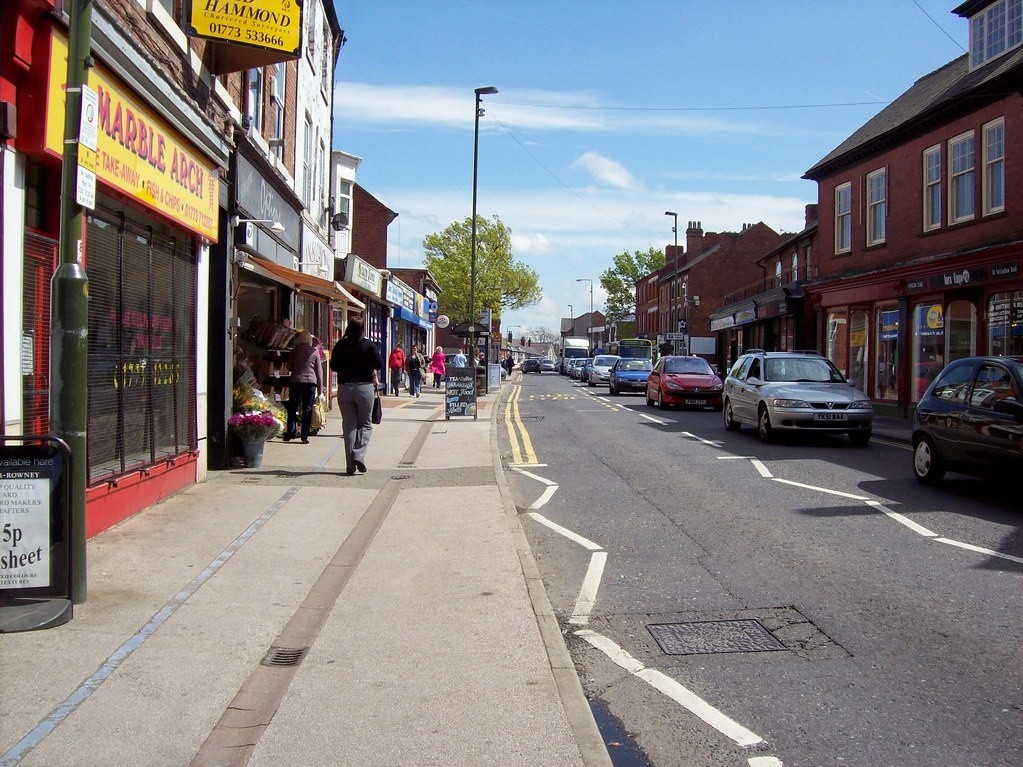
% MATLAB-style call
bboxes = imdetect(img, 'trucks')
[559,336,590,375]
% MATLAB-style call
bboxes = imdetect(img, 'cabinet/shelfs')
[263,348,293,401]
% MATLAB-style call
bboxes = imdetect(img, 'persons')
[501,355,514,376]
[388,342,406,396]
[418,344,429,384]
[405,345,425,397]
[454,350,468,368]
[329,316,383,474]
[430,346,446,388]
[759,362,778,380]
[474,349,485,366]
[283,331,323,443]
[578,362,583,365]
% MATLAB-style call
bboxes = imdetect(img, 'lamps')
[230,215,285,231]
[300,261,327,272]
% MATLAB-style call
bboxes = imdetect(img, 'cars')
[579,357,593,383]
[587,355,621,386]
[643,355,724,413]
[564,359,575,376]
[570,358,587,379]
[439,353,508,382]
[529,357,561,372]
[607,356,654,395]
[520,360,541,374]
[910,356,1023,491]
[87,347,176,384]
[915,361,942,402]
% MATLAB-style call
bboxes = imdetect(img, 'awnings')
[247,257,365,313]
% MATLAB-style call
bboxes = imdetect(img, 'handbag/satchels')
[310,396,325,428]
[372,388,382,424]
[419,368,426,378]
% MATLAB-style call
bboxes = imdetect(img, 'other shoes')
[302,439,309,443]
[283,432,295,441]
[354,461,366,473]
[416,393,420,398]
[396,392,398,396]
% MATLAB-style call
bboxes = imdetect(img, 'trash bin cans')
[477,366,487,396]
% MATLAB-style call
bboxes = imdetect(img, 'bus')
[616,339,653,365]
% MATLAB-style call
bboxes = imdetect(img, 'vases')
[243,441,263,467]
[309,428,319,435]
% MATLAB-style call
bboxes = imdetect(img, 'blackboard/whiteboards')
[445,366,477,416]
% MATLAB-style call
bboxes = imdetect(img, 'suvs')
[721,348,874,446]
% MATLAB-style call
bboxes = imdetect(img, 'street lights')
[507,326,522,341]
[664,210,678,355]
[567,303,575,336]
[576,279,594,358]
[470,86,498,368]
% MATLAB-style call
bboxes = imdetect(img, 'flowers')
[230,360,325,443]
